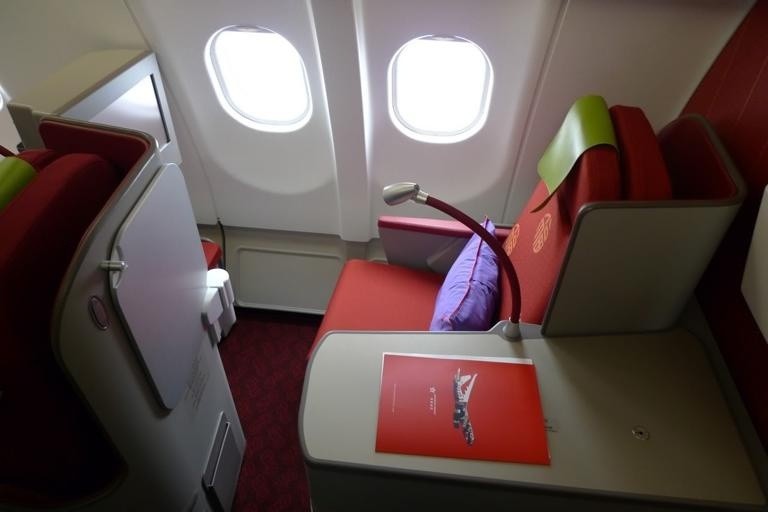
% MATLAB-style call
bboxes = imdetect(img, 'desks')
[297,325,768,511]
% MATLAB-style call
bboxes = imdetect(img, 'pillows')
[427,214,503,333]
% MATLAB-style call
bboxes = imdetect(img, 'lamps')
[382,180,522,338]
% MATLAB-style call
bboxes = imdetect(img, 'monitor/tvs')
[46,49,183,167]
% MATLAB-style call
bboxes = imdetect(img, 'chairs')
[304,97,671,358]
